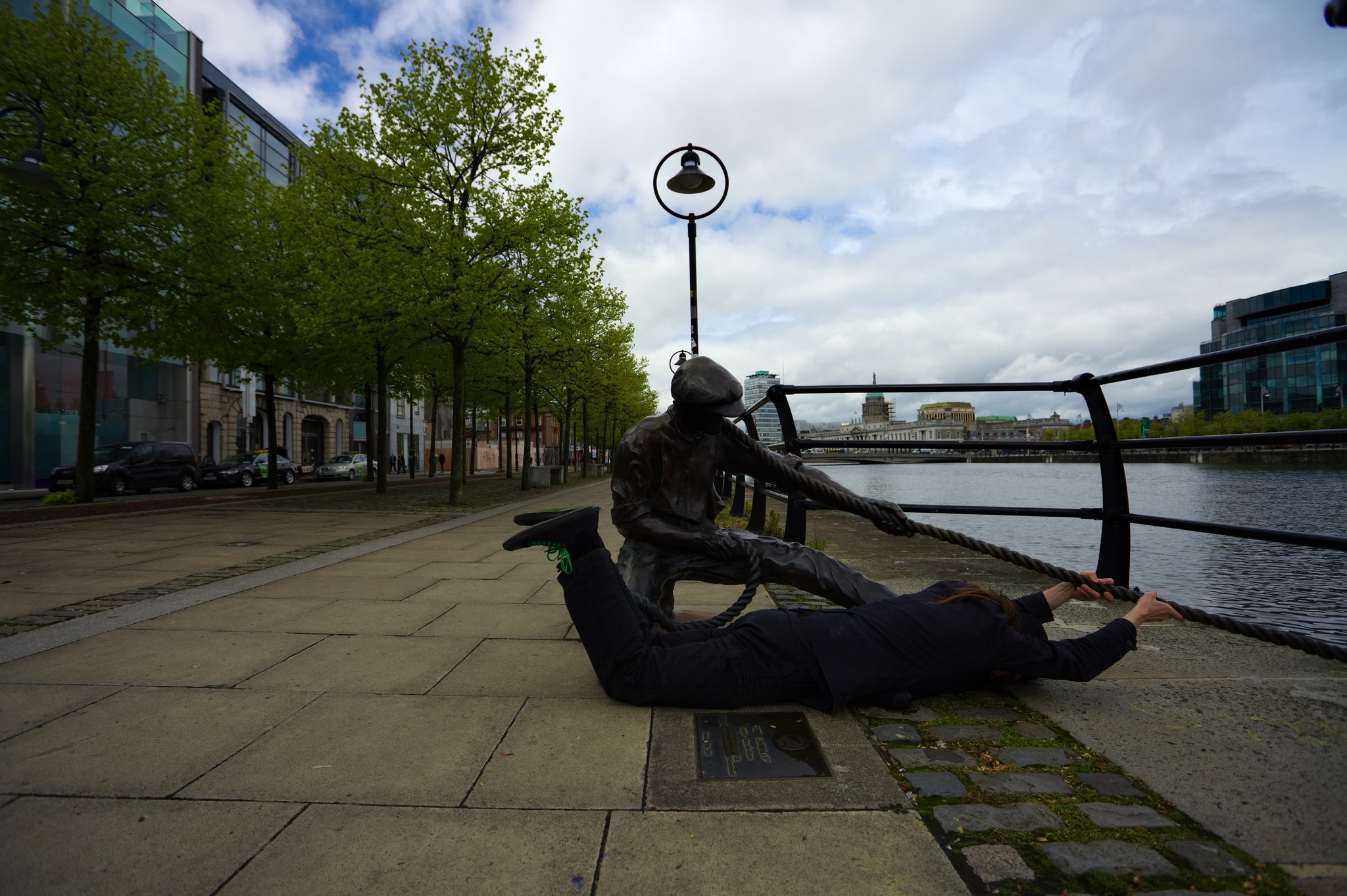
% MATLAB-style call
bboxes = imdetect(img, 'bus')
[827,449,845,454]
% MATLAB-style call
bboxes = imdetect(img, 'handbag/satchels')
[404,465,407,468]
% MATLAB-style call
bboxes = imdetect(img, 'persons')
[397,453,407,475]
[572,458,575,467]
[502,504,1188,712]
[608,355,906,616]
[590,446,597,464]
[438,452,445,473]
[530,455,532,466]
[568,458,571,467]
[389,452,397,474]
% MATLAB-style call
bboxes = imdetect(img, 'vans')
[912,448,932,454]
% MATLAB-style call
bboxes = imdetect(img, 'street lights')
[1260,386,1272,413]
[1116,401,1125,439]
[882,413,888,453]
[653,142,730,359]
[1026,412,1034,429]
[1076,413,1082,431]
[1334,386,1343,408]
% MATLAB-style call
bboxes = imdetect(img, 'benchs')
[581,463,604,477]
[607,463,611,473]
[530,466,564,487]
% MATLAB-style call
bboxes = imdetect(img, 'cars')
[201,446,300,489]
[814,450,825,454]
[965,451,975,455]
[314,451,378,481]
[996,449,1099,455]
[947,452,961,455]
[974,450,992,456]
[801,450,815,455]
[853,449,871,454]
[598,449,615,465]
[1305,443,1344,449]
[1268,444,1292,449]
[929,450,946,454]
[1121,447,1227,453]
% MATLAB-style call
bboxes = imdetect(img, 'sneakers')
[502,506,599,574]
[513,504,602,529]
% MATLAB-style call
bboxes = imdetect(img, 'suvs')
[48,441,203,497]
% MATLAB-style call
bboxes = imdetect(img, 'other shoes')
[403,473,406,475]
[389,472,391,474]
[397,473,399,474]
[395,472,396,474]
[441,470,444,472]
